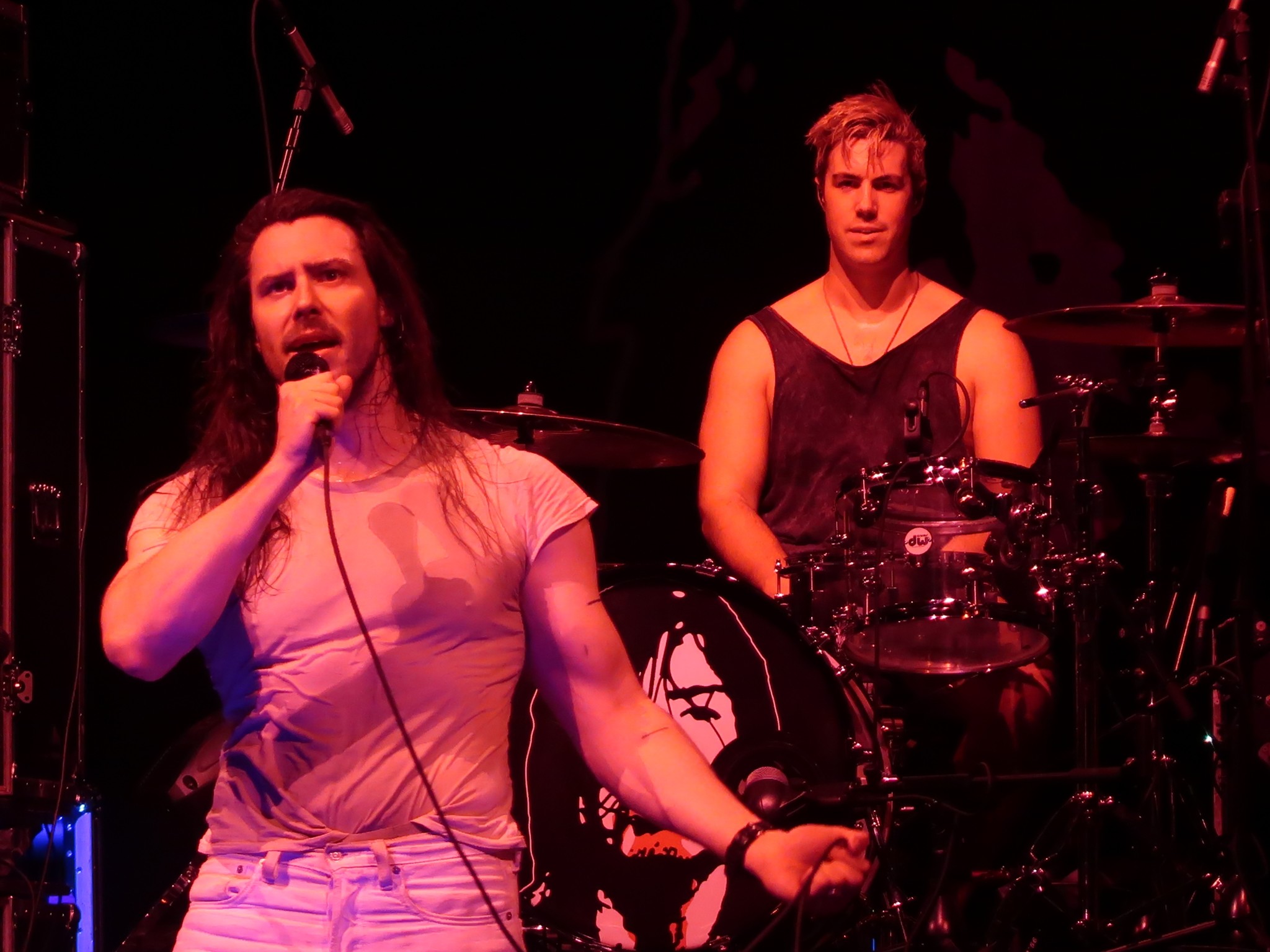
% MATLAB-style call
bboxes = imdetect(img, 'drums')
[832,456,1052,678]
[493,558,902,952]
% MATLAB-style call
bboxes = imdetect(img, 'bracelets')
[723,818,776,881]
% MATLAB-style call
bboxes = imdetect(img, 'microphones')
[271,0,354,135]
[285,351,333,445]
[917,381,934,456]
[1198,0,1244,94]
[744,767,817,826]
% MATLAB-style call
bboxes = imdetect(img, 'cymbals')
[1055,425,1246,474]
[1020,363,1156,409]
[440,401,708,465]
[998,298,1261,349]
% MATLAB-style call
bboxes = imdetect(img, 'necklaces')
[823,268,919,365]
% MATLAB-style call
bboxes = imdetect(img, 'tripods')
[977,312,1270,952]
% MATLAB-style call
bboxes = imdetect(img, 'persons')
[697,80,1057,765]
[101,189,881,952]
